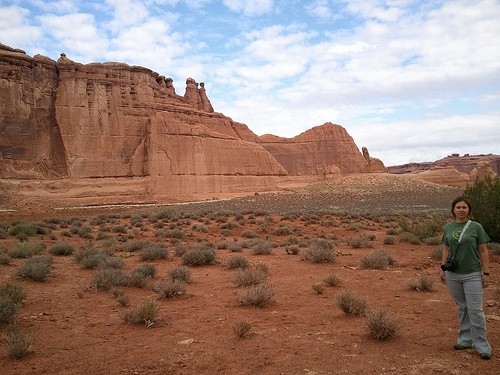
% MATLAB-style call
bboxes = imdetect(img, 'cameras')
[441,261,455,272]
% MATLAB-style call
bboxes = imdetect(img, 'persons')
[439,197,492,360]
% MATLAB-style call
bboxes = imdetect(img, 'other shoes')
[481,354,490,359]
[454,345,466,349]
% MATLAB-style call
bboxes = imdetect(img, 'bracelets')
[483,272,490,276]
